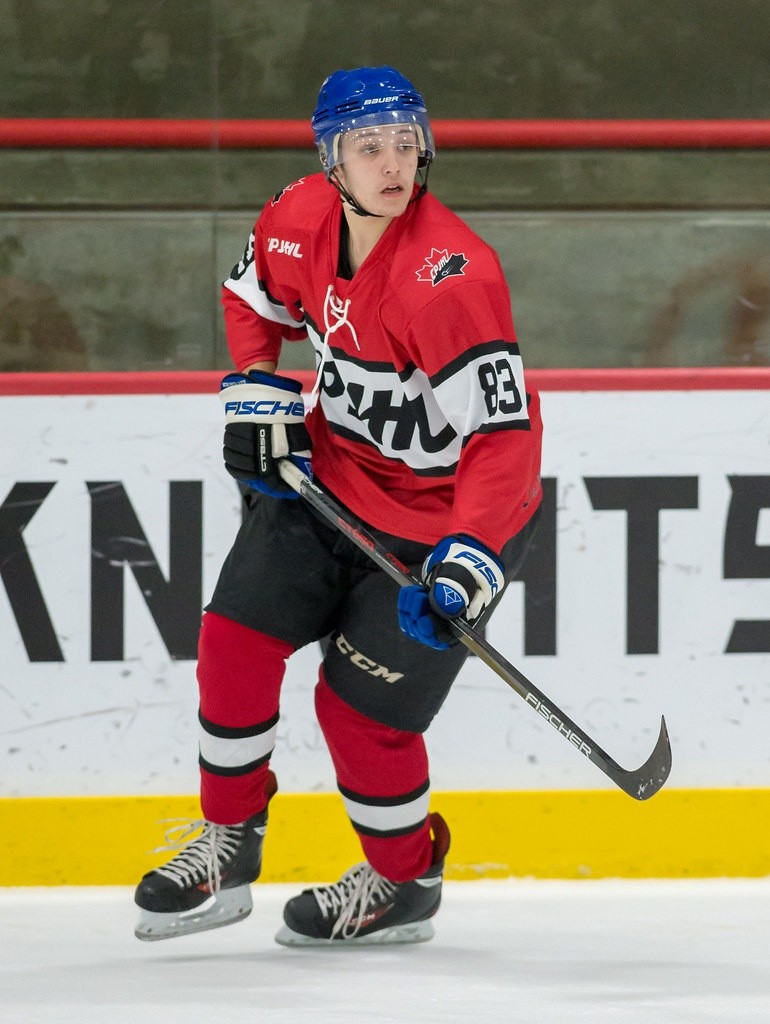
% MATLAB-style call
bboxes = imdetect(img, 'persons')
[132,65,543,947]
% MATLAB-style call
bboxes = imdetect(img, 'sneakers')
[276,810,452,949]
[134,767,278,942]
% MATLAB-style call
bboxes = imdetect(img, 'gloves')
[397,533,504,648]
[216,372,314,501]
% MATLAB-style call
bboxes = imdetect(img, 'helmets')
[312,65,434,180]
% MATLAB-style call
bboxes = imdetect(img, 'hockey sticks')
[270,455,676,805]
